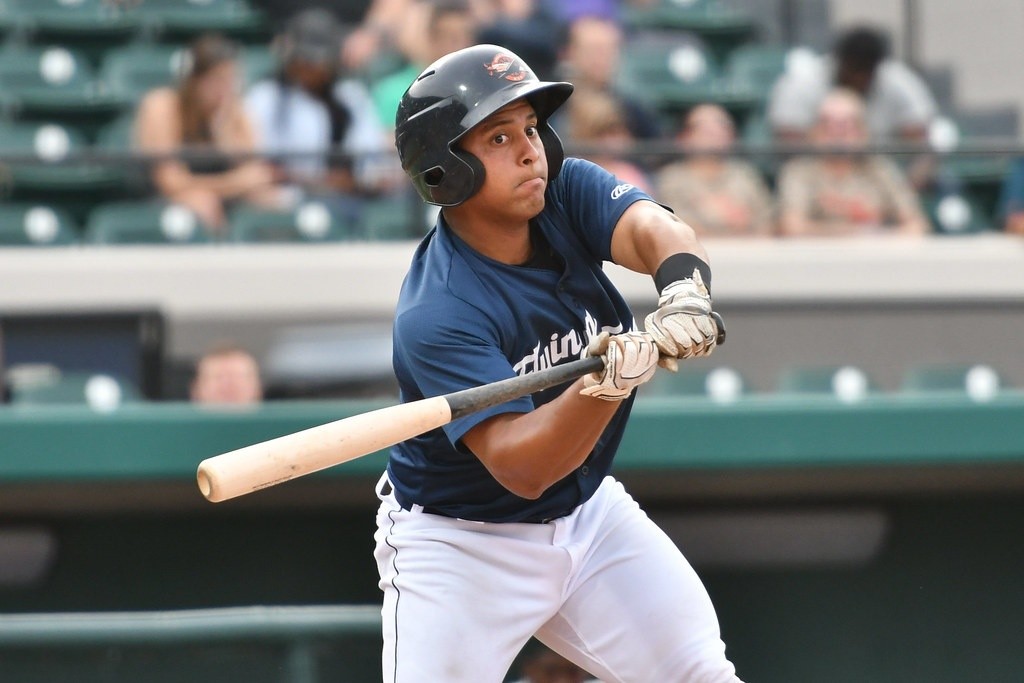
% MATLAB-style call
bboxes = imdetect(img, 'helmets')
[395,44,574,207]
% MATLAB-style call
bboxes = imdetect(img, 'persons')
[992,155,1024,234]
[127,0,954,238]
[374,41,752,681]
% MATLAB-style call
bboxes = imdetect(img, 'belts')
[393,487,555,523]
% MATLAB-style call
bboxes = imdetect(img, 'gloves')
[581,332,660,402]
[644,269,717,372]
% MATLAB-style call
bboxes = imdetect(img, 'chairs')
[0,0,1024,247]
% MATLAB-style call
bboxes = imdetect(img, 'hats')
[284,8,340,66]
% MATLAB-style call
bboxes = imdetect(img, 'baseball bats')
[198,310,730,505]
